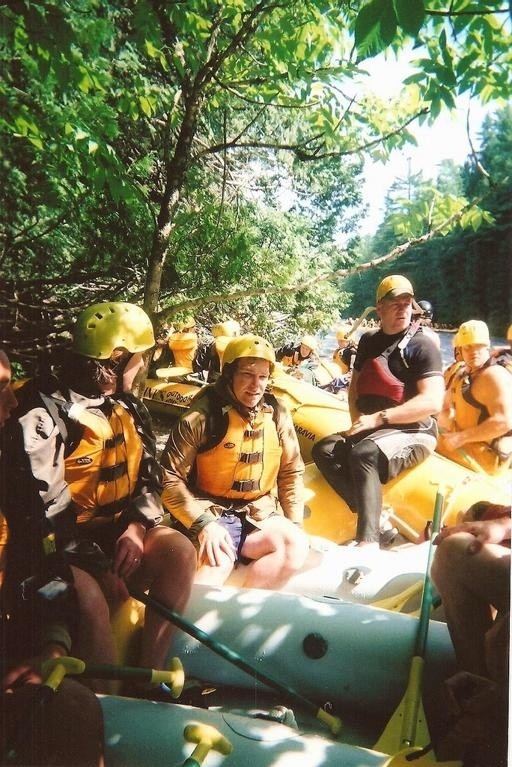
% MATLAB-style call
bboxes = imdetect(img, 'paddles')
[370,478,452,762]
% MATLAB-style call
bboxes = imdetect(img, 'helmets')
[67,301,155,360]
[452,319,491,347]
[416,299,433,319]
[301,336,316,351]
[336,330,349,341]
[213,319,275,373]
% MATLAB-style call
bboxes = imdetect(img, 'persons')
[2,274,512,767]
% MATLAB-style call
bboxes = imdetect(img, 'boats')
[137,350,510,625]
[91,582,457,767]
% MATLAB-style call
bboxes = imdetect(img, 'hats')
[377,275,414,303]
